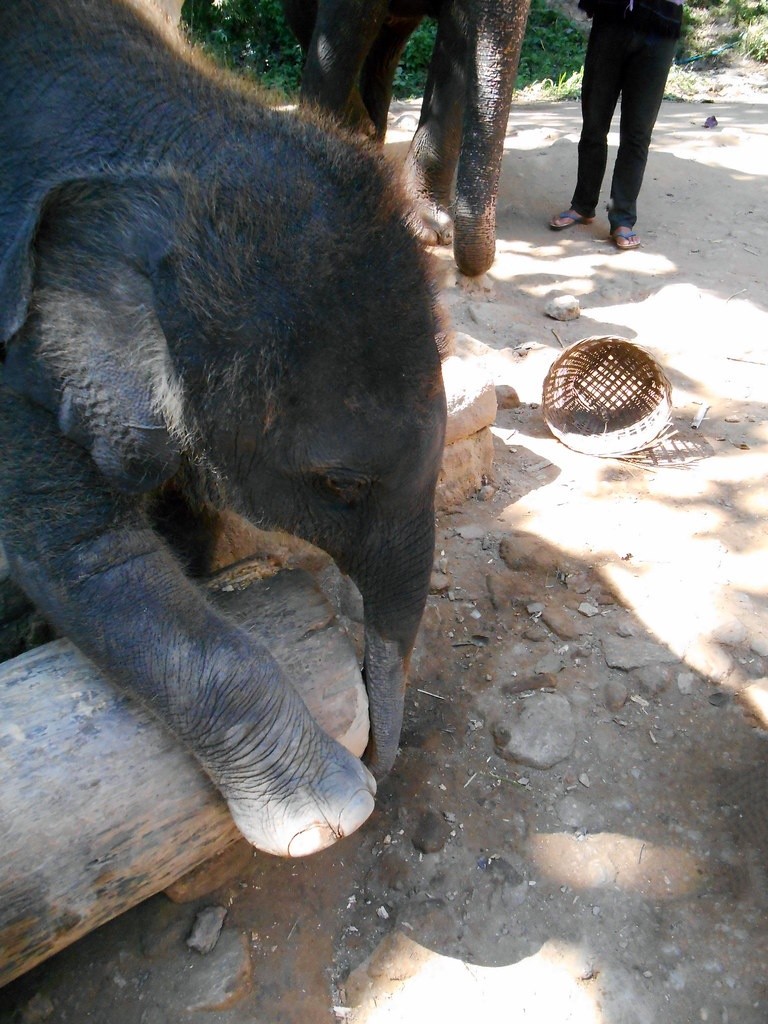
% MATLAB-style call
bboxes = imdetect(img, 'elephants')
[1,0,448,858]
[283,0,532,279]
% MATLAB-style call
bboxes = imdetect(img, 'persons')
[549,0,683,249]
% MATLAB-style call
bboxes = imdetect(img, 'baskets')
[542,334,679,461]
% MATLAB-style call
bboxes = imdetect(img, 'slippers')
[550,208,594,231]
[611,226,641,251]
